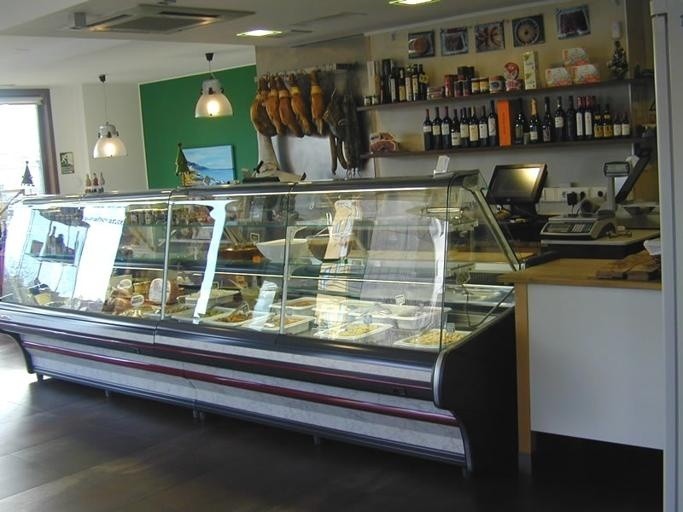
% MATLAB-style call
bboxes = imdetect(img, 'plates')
[514,18,540,45]
[559,9,590,38]
[441,32,467,56]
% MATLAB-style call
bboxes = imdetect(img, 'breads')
[102,277,179,312]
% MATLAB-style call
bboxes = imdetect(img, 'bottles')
[380,64,426,102]
[423,96,630,152]
[85,173,104,193]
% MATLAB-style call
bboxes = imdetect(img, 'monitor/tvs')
[485,163,547,203]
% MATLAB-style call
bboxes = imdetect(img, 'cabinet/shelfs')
[355,78,649,158]
[0,169,526,480]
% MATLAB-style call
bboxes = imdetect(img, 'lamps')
[194,52,233,118]
[93,74,128,158]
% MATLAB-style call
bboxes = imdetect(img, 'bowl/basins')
[623,206,655,216]
[255,236,353,264]
[239,288,278,309]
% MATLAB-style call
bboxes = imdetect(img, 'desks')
[540,227,660,259]
[497,258,664,475]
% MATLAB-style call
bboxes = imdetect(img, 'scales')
[540,161,631,240]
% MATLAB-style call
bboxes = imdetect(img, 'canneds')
[445,66,489,97]
